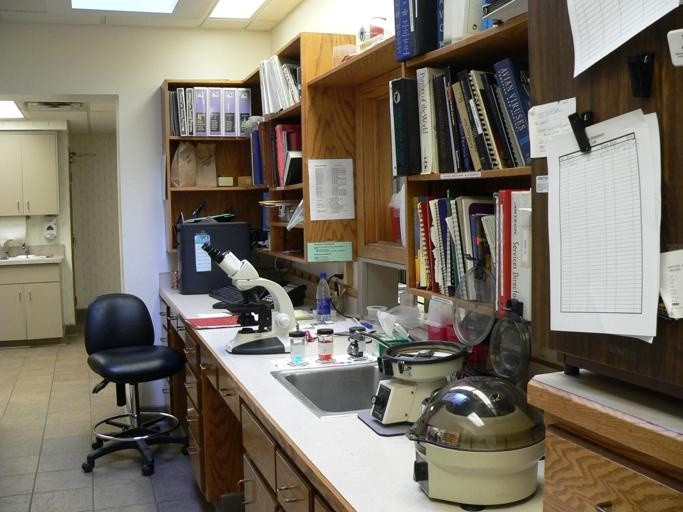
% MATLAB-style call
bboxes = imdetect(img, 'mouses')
[213,301,227,309]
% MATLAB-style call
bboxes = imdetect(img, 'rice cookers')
[406,375,547,512]
[368,265,531,426]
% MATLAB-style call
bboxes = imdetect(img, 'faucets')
[21,242,30,255]
[346,332,391,358]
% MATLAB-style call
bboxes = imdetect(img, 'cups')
[365,305,387,330]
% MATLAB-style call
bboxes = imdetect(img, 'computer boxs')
[175,221,249,294]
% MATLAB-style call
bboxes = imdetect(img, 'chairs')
[81,291,188,477]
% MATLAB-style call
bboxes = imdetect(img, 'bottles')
[288,331,306,365]
[314,272,332,324]
[316,328,334,363]
[349,327,367,360]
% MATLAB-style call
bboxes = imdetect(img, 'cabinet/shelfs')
[0,132,59,218]
[0,262,66,347]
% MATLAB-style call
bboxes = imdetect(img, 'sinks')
[8,254,46,261]
[270,362,397,419]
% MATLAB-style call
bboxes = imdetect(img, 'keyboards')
[209,285,248,303]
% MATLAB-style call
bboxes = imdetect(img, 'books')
[255,52,302,190]
[169,87,251,139]
[388,1,533,320]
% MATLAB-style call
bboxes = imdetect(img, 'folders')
[391,76,421,176]
